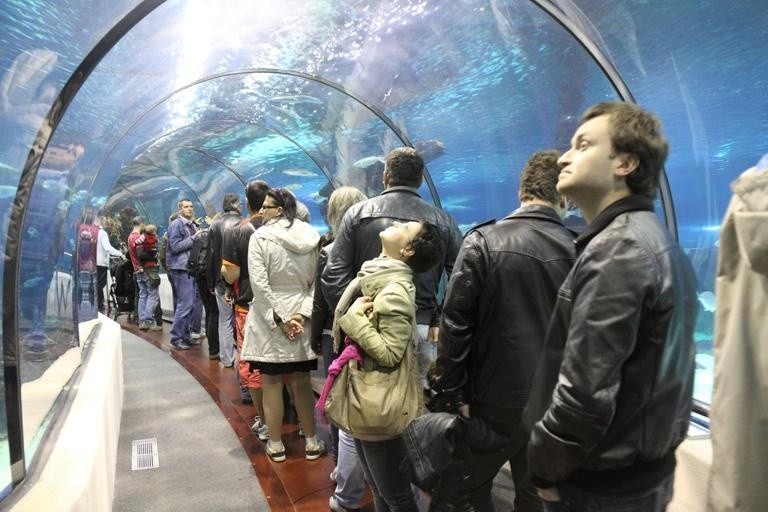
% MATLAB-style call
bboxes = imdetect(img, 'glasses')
[262,204,278,209]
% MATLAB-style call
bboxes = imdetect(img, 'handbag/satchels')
[148,272,160,287]
[325,345,420,439]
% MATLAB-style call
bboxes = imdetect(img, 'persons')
[75,198,209,351]
[240,188,327,461]
[221,179,308,440]
[706,151,768,512]
[316,217,444,512]
[428,149,586,511]
[206,192,247,367]
[321,146,465,512]
[293,201,312,221]
[522,102,698,512]
[308,186,370,480]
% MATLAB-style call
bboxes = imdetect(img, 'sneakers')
[171,331,207,350]
[305,440,327,461]
[265,440,286,462]
[251,420,269,441]
[330,496,348,512]
[208,354,233,367]
[139,323,162,331]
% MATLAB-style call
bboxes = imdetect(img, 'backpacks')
[185,239,210,278]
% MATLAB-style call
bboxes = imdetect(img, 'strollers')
[105,252,142,323]
